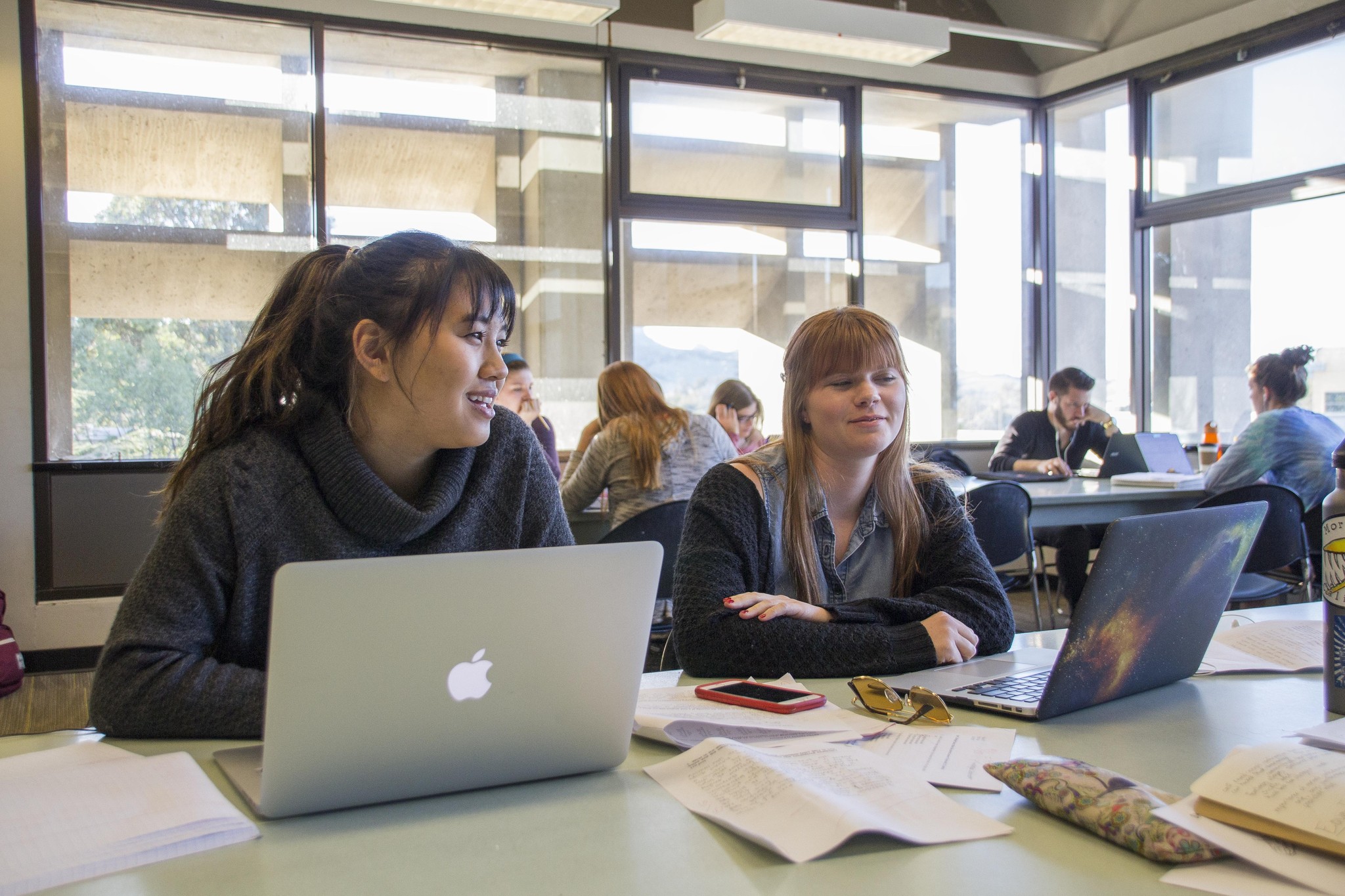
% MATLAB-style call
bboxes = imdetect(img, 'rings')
[1047,468,1053,476]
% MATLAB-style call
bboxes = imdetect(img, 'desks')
[0,600,1345,896]
[571,473,1217,544]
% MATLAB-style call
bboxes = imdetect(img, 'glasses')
[846,674,954,726]
[739,410,759,422]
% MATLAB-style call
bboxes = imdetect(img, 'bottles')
[1322,436,1345,717]
[1200,420,1222,460]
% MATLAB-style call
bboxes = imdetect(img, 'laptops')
[1073,432,1192,476]
[885,501,1269,721]
[212,540,664,820]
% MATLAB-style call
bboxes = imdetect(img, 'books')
[1111,472,1205,489]
[0,734,259,896]
[1188,740,1345,857]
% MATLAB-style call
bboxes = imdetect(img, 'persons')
[669,304,1019,681]
[87,230,577,740]
[987,366,1122,622]
[1202,345,1345,610]
[485,354,767,633]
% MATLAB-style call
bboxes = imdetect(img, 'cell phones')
[694,679,827,715]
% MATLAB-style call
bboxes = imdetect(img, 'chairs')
[959,481,1055,631]
[1187,483,1321,607]
[593,502,695,676]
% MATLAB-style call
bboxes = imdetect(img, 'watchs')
[1103,417,1117,429]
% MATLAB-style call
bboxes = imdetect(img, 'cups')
[1197,444,1219,472]
